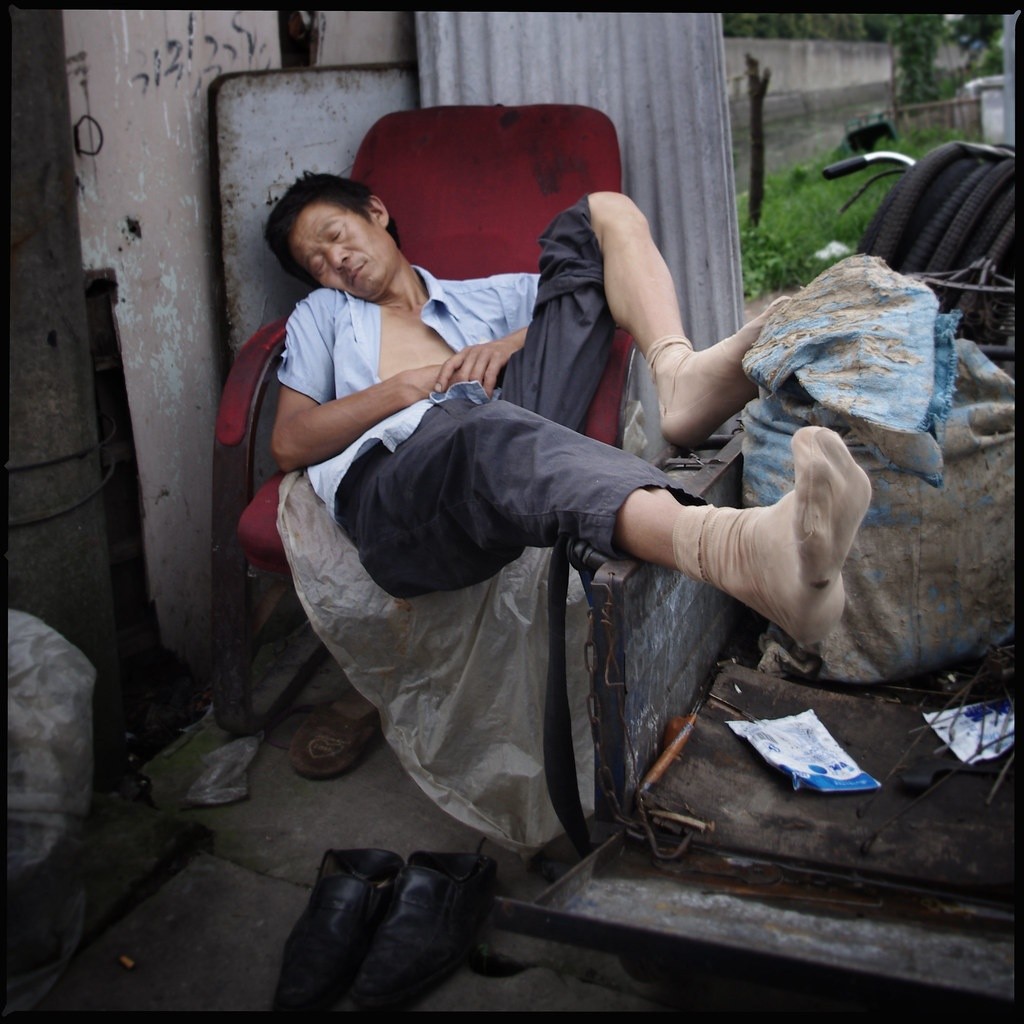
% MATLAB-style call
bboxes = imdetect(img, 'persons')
[263,172,874,650]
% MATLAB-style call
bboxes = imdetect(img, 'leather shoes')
[348,851,496,1012]
[269,848,404,1011]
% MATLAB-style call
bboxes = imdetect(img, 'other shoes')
[287,697,378,781]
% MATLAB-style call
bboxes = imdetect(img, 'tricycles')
[529,148,1014,1005]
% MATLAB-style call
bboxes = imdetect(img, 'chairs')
[207,104,637,735]
